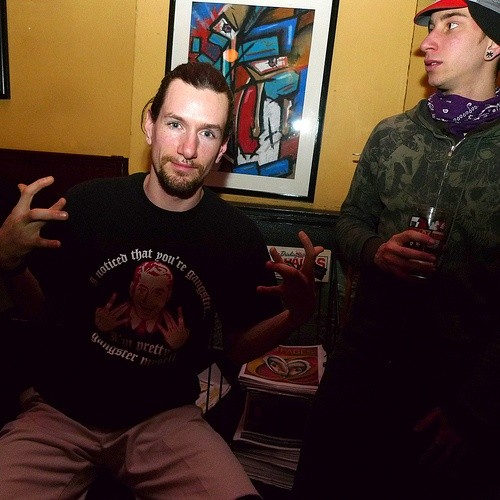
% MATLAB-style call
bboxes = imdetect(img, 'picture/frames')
[165,0,339,204]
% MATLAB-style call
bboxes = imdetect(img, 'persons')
[291,0,500,500]
[0,61,324,500]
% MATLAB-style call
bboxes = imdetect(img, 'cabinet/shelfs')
[199,244,342,500]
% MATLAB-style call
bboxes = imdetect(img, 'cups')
[403,205,453,279]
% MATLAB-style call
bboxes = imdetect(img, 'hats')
[413,0,500,45]
